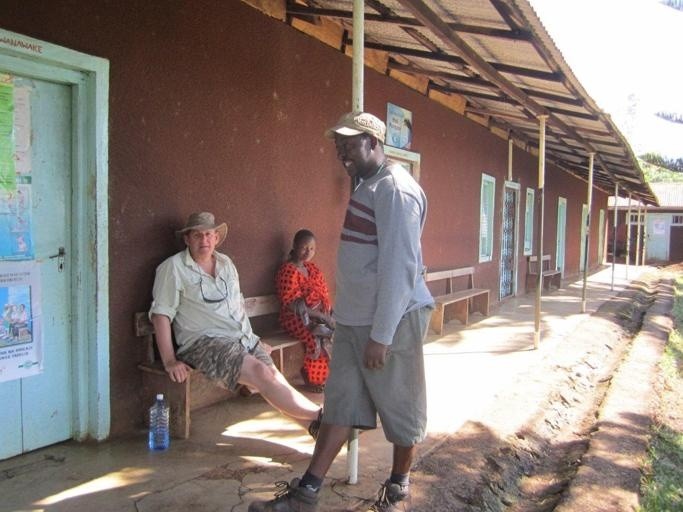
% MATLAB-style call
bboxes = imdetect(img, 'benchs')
[526,255,561,292]
[425,267,490,335]
[134,295,305,438]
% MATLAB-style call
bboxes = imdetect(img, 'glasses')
[199,277,228,302]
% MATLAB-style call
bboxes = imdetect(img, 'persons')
[148,212,323,441]
[247,111,433,508]
[276,229,335,392]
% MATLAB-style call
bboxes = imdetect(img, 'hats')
[174,211,228,249]
[324,111,387,144]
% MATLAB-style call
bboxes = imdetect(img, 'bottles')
[148,393,169,449]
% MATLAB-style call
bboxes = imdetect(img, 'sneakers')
[248,477,321,512]
[365,479,410,512]
